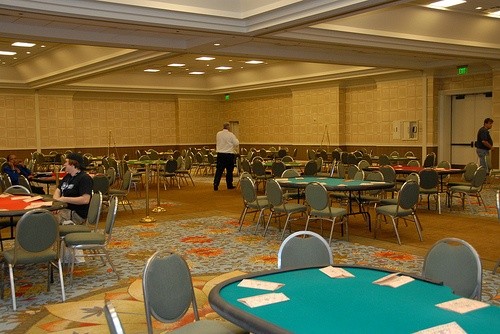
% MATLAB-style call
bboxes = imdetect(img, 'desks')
[270,174,395,237]
[371,163,466,211]
[29,176,62,194]
[208,264,500,334]
[125,159,167,186]
[0,191,68,238]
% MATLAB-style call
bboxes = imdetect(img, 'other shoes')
[214,184,217,191]
[227,186,236,189]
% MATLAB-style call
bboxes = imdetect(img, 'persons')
[53,153,93,266]
[476,118,494,171]
[214,122,239,191]
[1,153,45,195]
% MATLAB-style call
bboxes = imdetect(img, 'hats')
[65,153,83,165]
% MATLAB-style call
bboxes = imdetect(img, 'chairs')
[0,141,489,334]
